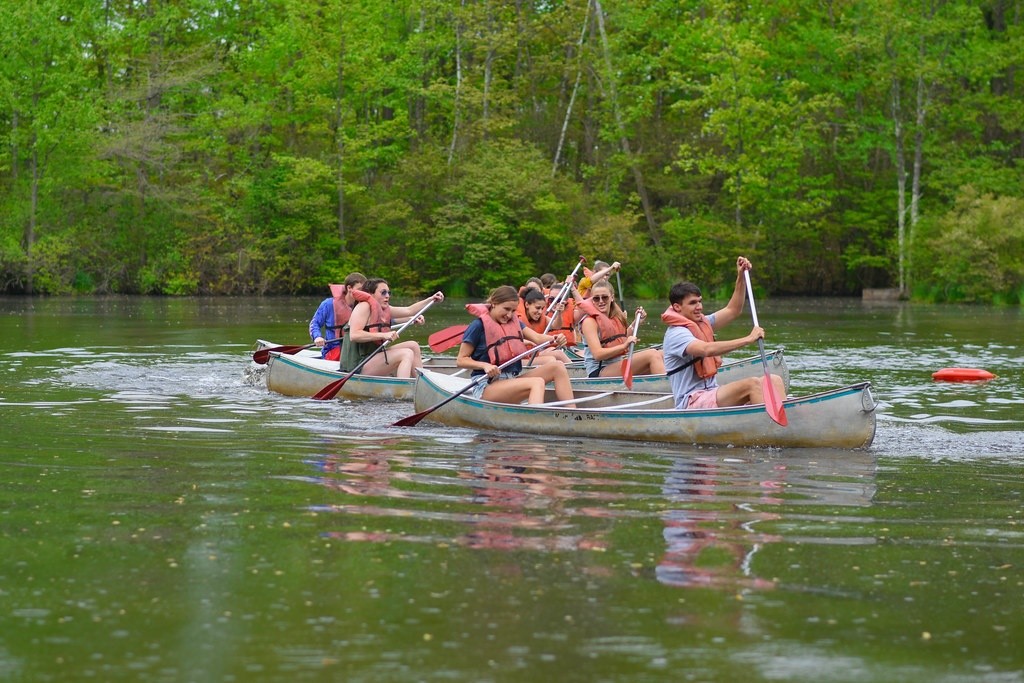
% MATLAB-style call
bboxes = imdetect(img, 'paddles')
[740,259,790,426]
[619,306,644,391]
[391,335,568,426]
[545,279,565,297]
[252,320,418,364]
[614,265,627,313]
[312,293,443,400]
[545,253,587,316]
[528,273,578,367]
[426,323,470,353]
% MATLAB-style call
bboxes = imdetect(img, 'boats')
[256,339,483,406]
[415,347,792,399]
[413,367,877,455]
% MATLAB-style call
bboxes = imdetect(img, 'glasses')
[592,295,611,301]
[548,298,562,303]
[378,291,391,296]
[604,274,611,276]
[544,286,551,289]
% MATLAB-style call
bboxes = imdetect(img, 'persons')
[456,285,579,409]
[580,281,666,378]
[511,286,577,366]
[662,255,790,411]
[512,260,623,351]
[309,272,368,360]
[340,278,425,379]
